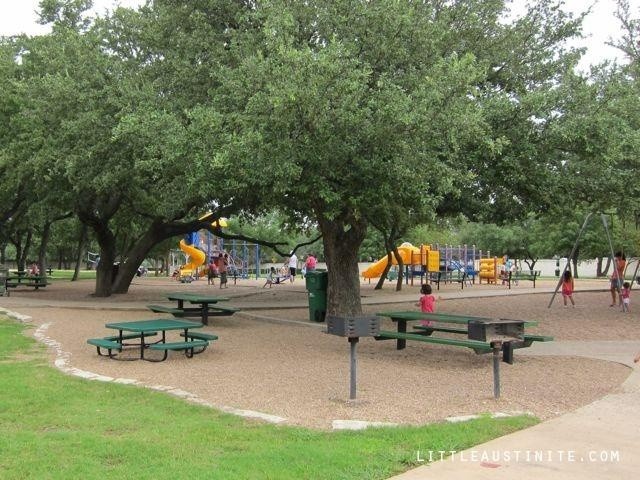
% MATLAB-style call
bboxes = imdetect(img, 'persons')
[26,260,40,280]
[501,254,519,287]
[204,249,317,291]
[608,250,626,309]
[413,283,437,327]
[622,283,631,314]
[562,271,577,310]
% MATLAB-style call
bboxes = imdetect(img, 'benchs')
[371,334,492,356]
[7,271,52,290]
[501,271,540,289]
[86,291,242,363]
[413,323,553,348]
[429,270,466,290]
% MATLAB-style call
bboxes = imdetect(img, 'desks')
[374,309,526,343]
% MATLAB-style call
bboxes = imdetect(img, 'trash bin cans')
[305,269,327,321]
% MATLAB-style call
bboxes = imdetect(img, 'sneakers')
[571,305,576,310]
[563,305,567,309]
